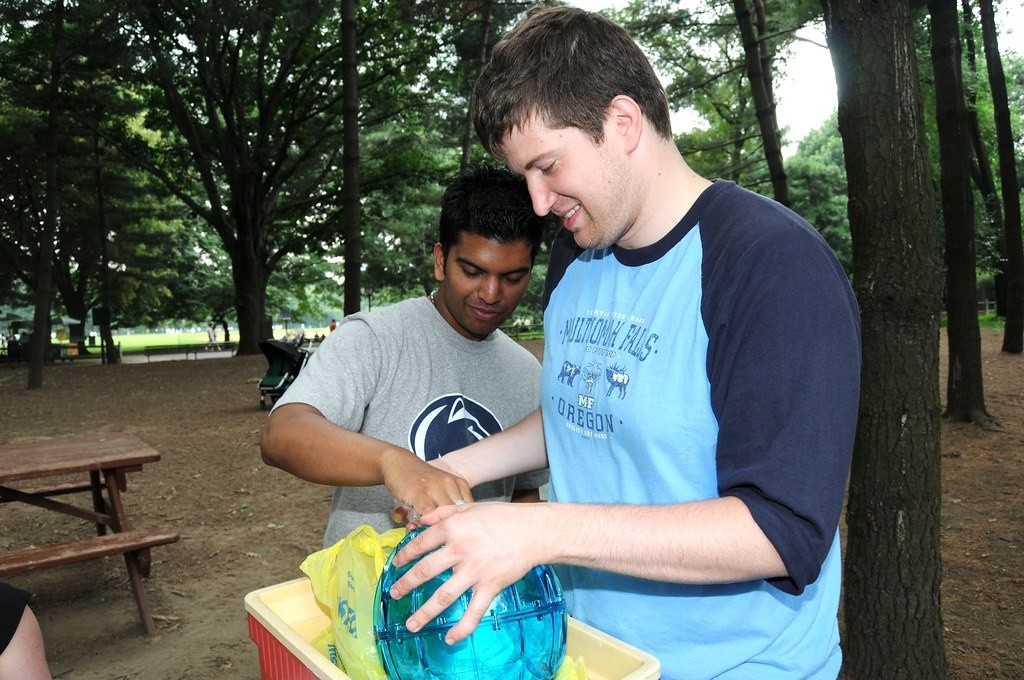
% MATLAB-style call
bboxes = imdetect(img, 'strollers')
[256,331,315,411]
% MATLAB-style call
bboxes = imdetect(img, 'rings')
[457,501,465,504]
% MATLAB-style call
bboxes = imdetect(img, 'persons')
[315,332,325,341]
[205,323,222,352]
[388,3,862,680]
[0,582,52,680]
[260,170,550,550]
[330,319,335,332]
[2,334,7,348]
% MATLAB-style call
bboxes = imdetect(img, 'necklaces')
[430,291,436,304]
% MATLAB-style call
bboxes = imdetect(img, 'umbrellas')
[52,317,82,325]
[0,313,21,335]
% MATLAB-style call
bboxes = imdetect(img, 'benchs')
[0,475,106,504]
[0,525,179,578]
[144,342,237,363]
[50,342,122,365]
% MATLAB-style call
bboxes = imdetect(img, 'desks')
[0,432,162,636]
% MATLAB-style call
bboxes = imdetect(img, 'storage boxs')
[244,570,663,680]
